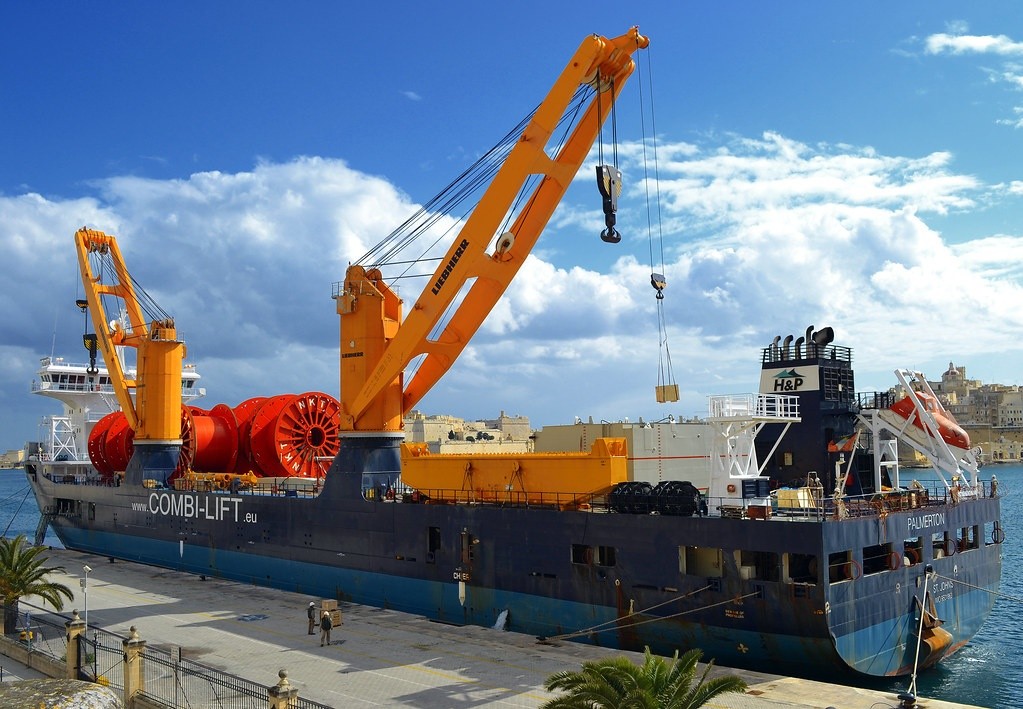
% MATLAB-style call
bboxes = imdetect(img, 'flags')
[828,432,856,452]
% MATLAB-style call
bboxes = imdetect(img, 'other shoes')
[309,633,311,634]
[311,633,315,635]
[321,644,324,647]
[327,643,330,645]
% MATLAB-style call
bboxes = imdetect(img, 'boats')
[17,327,1006,687]
[878,391,970,447]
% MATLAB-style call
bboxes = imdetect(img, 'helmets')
[992,475,996,478]
[323,611,329,615]
[310,602,315,607]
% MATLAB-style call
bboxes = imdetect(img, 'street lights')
[82,565,92,659]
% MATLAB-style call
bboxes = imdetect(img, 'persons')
[319,611,333,646]
[307,601,316,635]
[832,488,840,505]
[990,475,998,498]
[911,479,924,489]
[115,473,120,487]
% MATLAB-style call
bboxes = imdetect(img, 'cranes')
[304,25,684,509]
[77,227,260,493]
